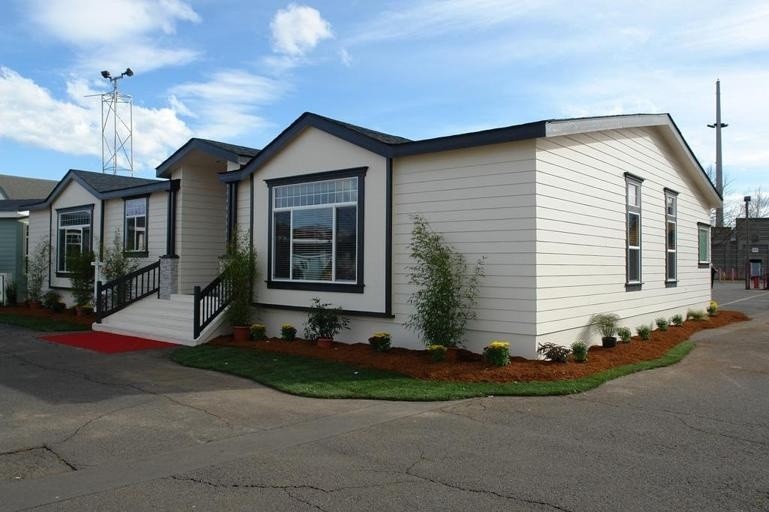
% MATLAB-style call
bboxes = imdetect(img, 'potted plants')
[303,297,353,349]
[401,211,488,363]
[5,226,147,320]
[590,311,621,348]
[214,223,258,343]
[570,342,588,362]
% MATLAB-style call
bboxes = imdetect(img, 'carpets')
[39,331,181,355]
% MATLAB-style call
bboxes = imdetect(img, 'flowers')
[249,323,295,343]
[368,331,512,368]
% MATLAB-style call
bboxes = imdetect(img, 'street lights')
[743,196,752,290]
[101,68,134,175]
